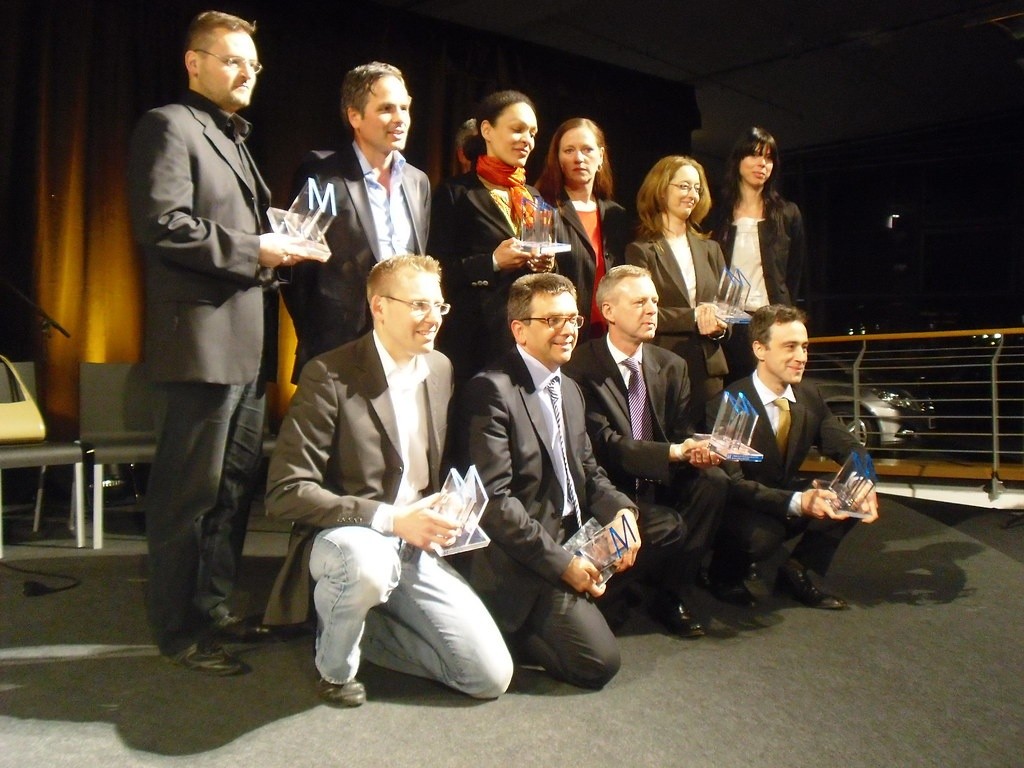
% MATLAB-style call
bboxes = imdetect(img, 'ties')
[774,398,792,459]
[622,358,656,515]
[549,376,582,531]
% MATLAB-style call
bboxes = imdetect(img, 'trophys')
[812,451,876,519]
[565,513,638,588]
[268,176,337,263]
[510,198,573,255]
[692,391,765,463]
[432,463,489,558]
[699,267,754,324]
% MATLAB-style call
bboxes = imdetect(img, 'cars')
[794,346,937,458]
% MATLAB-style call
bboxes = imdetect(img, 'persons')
[268,61,807,588]
[563,264,734,640]
[123,11,332,677]
[442,272,642,691]
[263,253,516,708]
[704,305,881,608]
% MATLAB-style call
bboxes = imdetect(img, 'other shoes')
[171,637,243,676]
[207,618,279,641]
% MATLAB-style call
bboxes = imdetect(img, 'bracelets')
[675,444,686,462]
[492,253,501,272]
[708,330,726,341]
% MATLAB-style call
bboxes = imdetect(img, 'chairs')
[66,362,158,551]
[0,361,86,560]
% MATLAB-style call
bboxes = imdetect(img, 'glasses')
[192,48,263,75]
[518,316,584,328]
[380,295,451,316]
[668,182,705,194]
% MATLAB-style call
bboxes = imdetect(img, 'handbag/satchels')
[0,356,45,443]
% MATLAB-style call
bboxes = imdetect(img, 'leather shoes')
[314,665,366,708]
[696,576,760,611]
[777,566,848,609]
[650,595,705,637]
